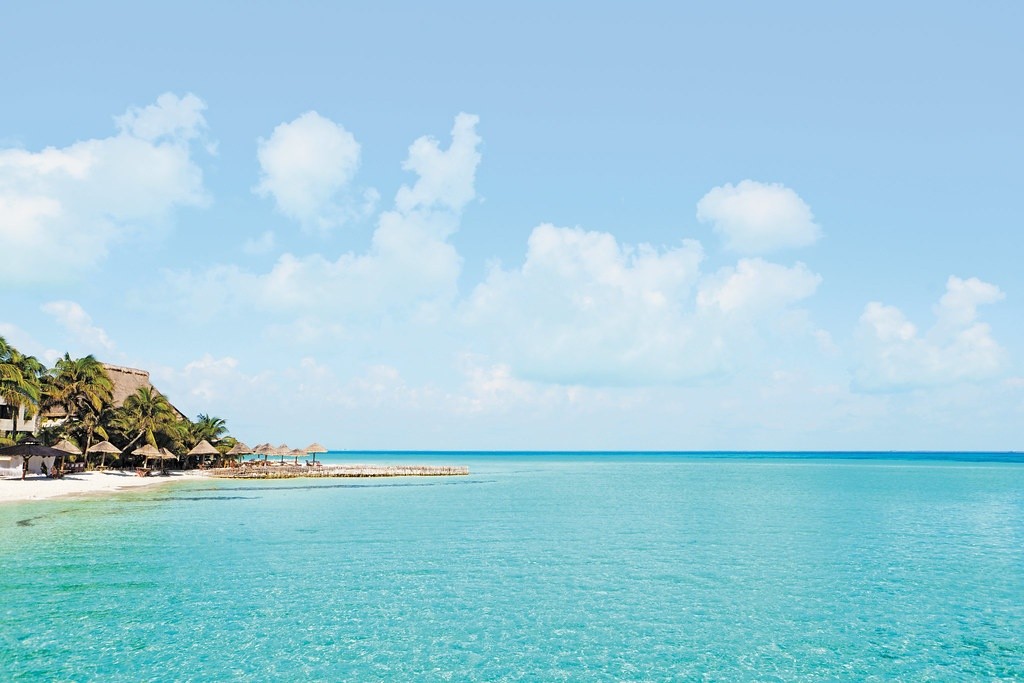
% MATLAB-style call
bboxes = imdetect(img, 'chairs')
[197,460,323,471]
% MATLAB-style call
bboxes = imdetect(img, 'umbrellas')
[0,438,330,478]
